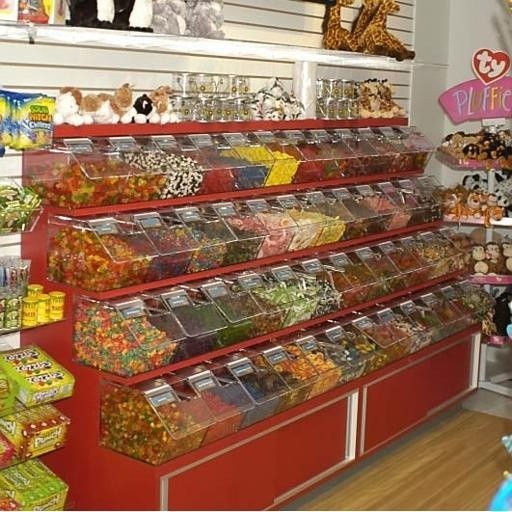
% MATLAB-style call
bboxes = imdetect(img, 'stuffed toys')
[484,242,507,275]
[360,0,416,62]
[121,0,153,32]
[438,128,512,169]
[153,0,187,36]
[500,241,512,276]
[441,170,512,228]
[186,0,224,40]
[471,243,489,275]
[68,0,117,30]
[352,0,375,38]
[54,80,180,126]
[321,0,360,53]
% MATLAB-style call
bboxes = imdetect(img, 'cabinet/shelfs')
[20,113,489,503]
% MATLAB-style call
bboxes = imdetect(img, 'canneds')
[0,285,66,331]
